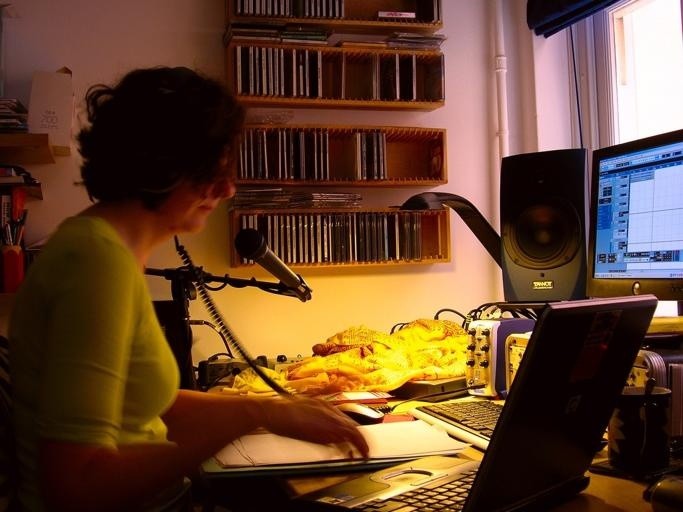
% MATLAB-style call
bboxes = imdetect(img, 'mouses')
[335,403,384,425]
[648,475,682,511]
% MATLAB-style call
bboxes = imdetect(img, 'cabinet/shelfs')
[0,179,44,201]
[231,210,452,265]
[226,39,446,112]
[0,134,56,165]
[224,0,443,48]
[233,124,449,187]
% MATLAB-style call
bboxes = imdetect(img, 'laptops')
[295,295,658,512]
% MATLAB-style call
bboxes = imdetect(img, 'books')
[195,415,471,476]
[239,126,330,180]
[351,131,387,181]
[236,0,345,19]
[235,44,323,99]
[371,51,380,100]
[332,50,346,100]
[376,11,416,20]
[437,213,442,259]
[393,53,400,100]
[0,99,28,129]
[412,55,416,101]
[232,187,363,209]
[223,22,328,46]
[240,212,422,264]
[327,28,447,50]
[0,167,25,184]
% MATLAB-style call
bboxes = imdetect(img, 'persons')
[5,64,247,512]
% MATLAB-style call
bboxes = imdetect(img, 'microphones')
[237,227,302,289]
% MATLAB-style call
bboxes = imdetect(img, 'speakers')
[500,147,587,303]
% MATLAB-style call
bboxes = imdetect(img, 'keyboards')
[415,396,504,451]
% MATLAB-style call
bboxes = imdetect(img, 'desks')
[284,387,683,511]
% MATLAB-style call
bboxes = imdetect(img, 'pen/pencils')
[4,208,28,246]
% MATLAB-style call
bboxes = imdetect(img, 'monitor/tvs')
[587,130,683,304]
